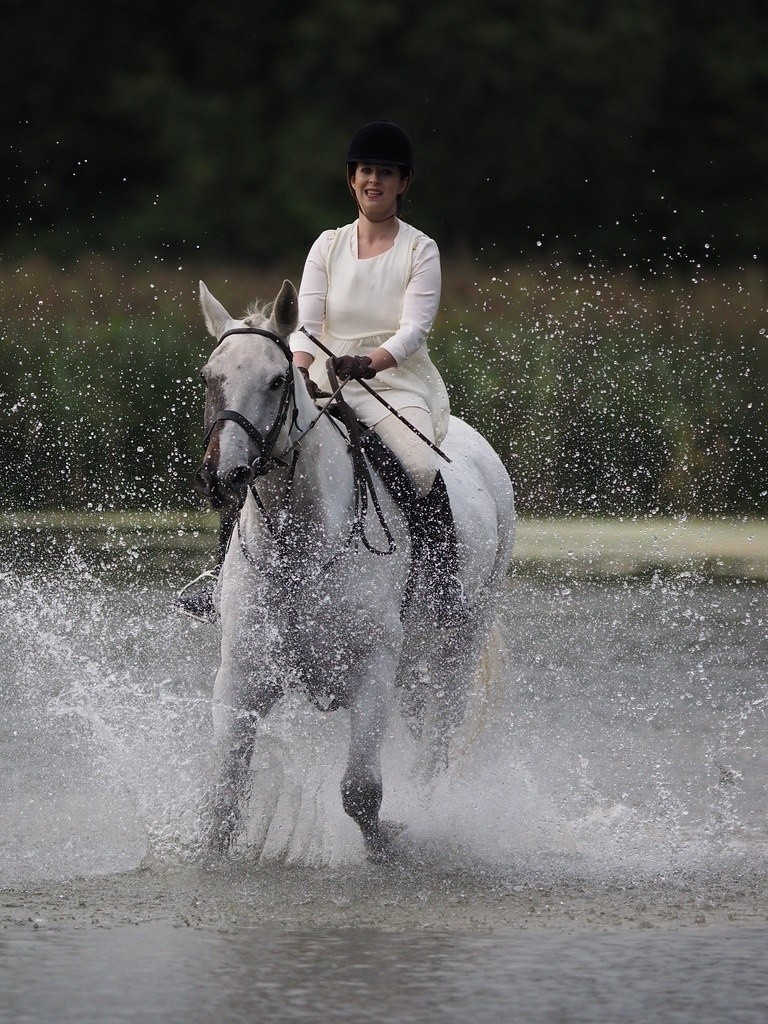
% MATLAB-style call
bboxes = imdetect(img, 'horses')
[195,280,517,871]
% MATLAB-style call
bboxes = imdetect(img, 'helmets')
[345,116,414,167]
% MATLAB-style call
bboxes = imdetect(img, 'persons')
[179,122,468,629]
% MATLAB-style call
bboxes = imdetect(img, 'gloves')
[333,355,376,382]
[297,367,321,402]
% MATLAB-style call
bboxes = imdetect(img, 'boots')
[175,511,238,618]
[410,469,467,629]
